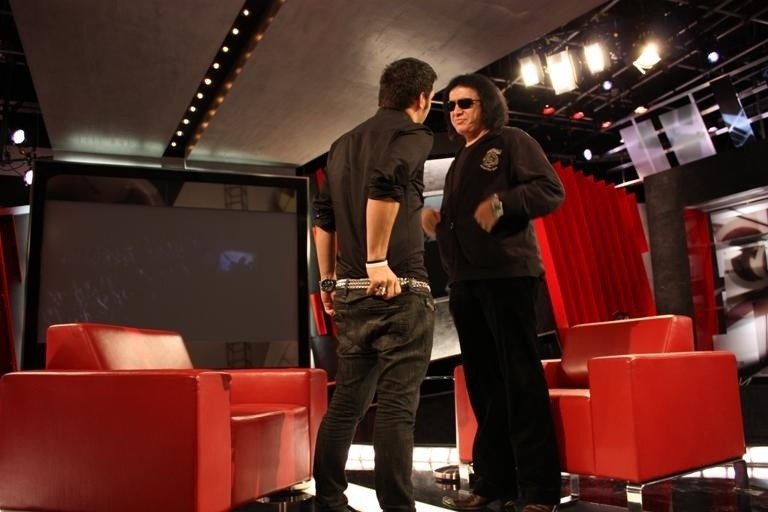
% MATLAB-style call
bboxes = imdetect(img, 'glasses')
[447,98,479,111]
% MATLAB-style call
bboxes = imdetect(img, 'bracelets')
[365,259,388,268]
[490,193,504,218]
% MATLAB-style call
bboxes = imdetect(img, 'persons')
[311,58,436,512]
[420,73,566,512]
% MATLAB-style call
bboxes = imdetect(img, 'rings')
[379,287,385,295]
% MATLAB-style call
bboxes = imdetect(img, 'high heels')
[522,504,557,512]
[442,489,518,510]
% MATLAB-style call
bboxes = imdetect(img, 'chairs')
[449,314,754,511]
[1,318,330,512]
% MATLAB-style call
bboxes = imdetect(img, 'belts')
[335,277,429,289]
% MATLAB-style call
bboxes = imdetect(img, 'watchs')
[318,279,336,293]
